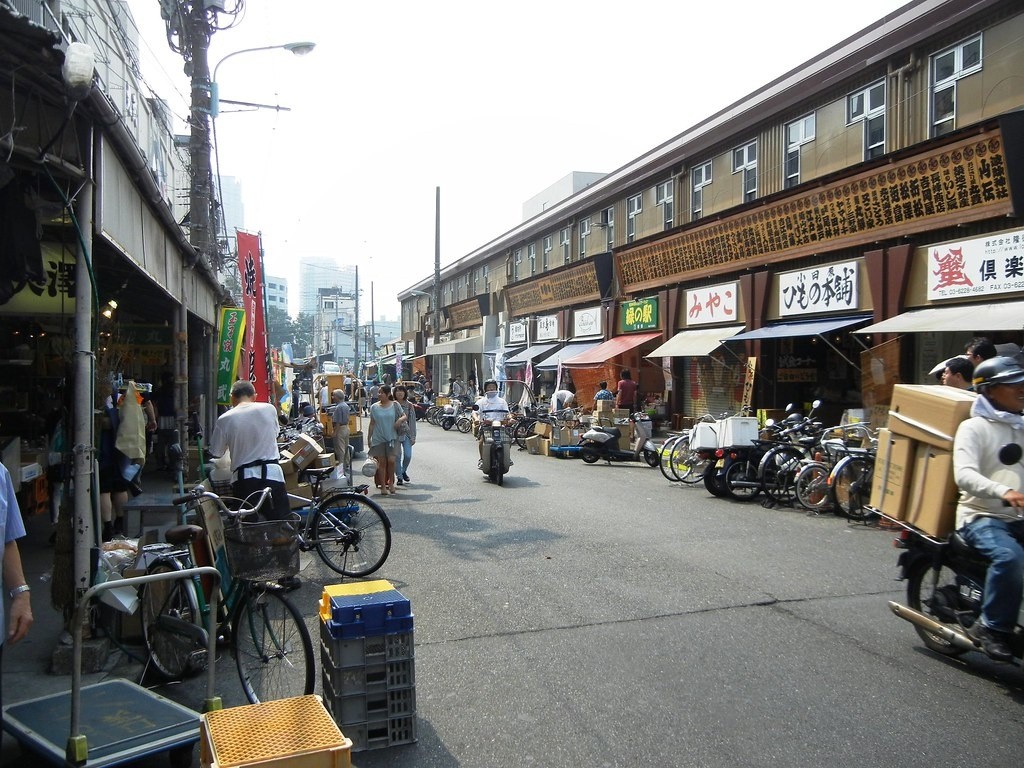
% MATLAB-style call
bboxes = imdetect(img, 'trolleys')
[2,566,223,768]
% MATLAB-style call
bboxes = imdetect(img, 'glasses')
[965,353,973,355]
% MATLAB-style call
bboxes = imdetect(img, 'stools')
[671,413,696,431]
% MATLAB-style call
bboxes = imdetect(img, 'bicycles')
[139,487,314,705]
[657,404,755,487]
[424,393,583,449]
[278,404,329,453]
[212,465,392,584]
[748,416,880,525]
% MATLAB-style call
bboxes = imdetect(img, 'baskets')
[224,520,300,582]
[483,428,513,444]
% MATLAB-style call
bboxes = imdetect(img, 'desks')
[121,493,190,538]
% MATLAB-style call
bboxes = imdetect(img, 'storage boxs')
[436,397,450,407]
[20,452,43,483]
[279,432,348,508]
[525,399,652,457]
[848,408,871,441]
[188,446,201,481]
[319,579,419,752]
[836,485,858,513]
[116,564,177,639]
[757,409,802,440]
[717,417,759,448]
[472,423,479,435]
[198,694,353,768]
[320,412,358,436]
[871,384,980,537]
[689,422,717,451]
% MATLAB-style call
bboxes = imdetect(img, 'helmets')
[787,413,803,423]
[972,355,1024,395]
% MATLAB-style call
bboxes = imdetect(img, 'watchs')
[9,585,29,600]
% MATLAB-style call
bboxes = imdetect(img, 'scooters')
[408,393,438,421]
[440,397,472,431]
[577,410,659,469]
[694,397,823,498]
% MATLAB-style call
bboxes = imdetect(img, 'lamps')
[603,295,658,311]
[521,314,538,325]
[39,42,94,158]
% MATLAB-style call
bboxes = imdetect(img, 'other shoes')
[278,578,301,589]
[968,618,1014,661]
[381,488,388,494]
[477,460,483,469]
[114,517,124,532]
[402,472,410,481]
[129,480,143,497]
[102,521,115,541]
[388,480,395,493]
[509,459,514,465]
[397,479,403,485]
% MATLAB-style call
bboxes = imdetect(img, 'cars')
[321,360,340,374]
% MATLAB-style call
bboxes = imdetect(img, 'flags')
[214,229,271,406]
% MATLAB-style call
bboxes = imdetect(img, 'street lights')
[409,288,439,397]
[186,42,315,253]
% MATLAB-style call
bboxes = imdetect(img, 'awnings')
[382,355,413,365]
[482,313,875,381]
[426,335,483,355]
[849,300,1024,384]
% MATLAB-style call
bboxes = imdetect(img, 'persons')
[298,389,351,472]
[0,462,34,754]
[47,379,157,546]
[552,390,577,410]
[592,370,636,443]
[367,385,416,495]
[941,336,1024,660]
[472,379,515,469]
[208,380,302,593]
[292,369,476,421]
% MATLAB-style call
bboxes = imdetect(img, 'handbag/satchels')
[362,458,378,477]
[394,401,410,435]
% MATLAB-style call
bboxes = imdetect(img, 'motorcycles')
[861,444,1024,667]
[472,403,523,486]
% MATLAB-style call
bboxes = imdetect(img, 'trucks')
[292,366,314,395]
